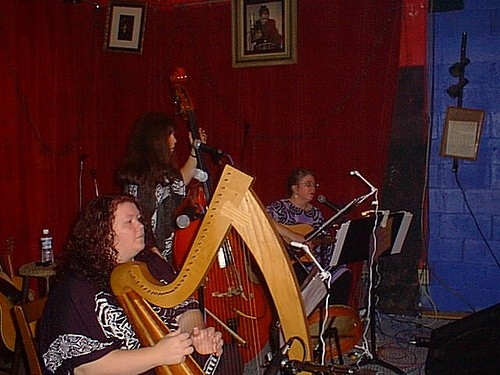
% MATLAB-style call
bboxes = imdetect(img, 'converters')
[416,337,430,347]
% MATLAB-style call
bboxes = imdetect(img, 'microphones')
[318,195,340,213]
[263,340,294,375]
[177,204,201,227]
[313,331,330,358]
[193,140,219,154]
[193,169,209,181]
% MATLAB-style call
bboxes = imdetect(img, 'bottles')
[40,229,54,264]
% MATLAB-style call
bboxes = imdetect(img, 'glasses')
[295,183,319,188]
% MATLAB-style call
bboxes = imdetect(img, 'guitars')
[278,223,338,263]
[0,256,39,357]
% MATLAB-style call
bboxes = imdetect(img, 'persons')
[115,109,207,261]
[264,168,352,307]
[31,194,223,375]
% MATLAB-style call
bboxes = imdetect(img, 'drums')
[308,302,362,359]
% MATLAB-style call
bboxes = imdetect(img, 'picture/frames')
[102,0,149,55]
[231,0,298,68]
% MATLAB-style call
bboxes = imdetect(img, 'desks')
[12,262,56,375]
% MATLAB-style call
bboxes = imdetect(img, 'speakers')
[426,304,500,375]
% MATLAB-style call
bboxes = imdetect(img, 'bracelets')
[190,153,197,158]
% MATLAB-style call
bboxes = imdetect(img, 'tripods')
[335,212,406,374]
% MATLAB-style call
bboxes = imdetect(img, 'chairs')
[14,296,49,375]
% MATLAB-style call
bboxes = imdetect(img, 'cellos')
[170,68,273,364]
